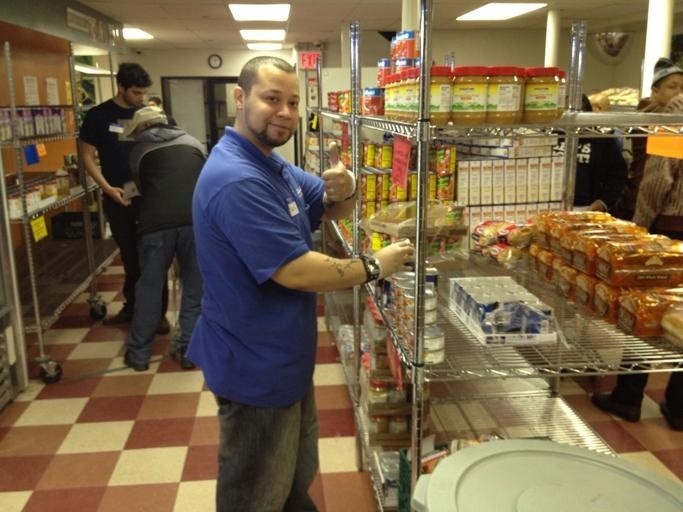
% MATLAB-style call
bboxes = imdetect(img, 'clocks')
[208,53,222,69]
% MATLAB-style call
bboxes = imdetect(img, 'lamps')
[240,28,285,42]
[247,43,282,51]
[228,2,290,22]
[111,28,152,41]
[455,2,547,22]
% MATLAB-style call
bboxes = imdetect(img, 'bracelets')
[344,169,357,200]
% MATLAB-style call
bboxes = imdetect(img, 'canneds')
[376,260,445,366]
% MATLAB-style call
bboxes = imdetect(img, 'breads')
[529,243,540,270]
[535,212,612,246]
[593,284,620,325]
[558,265,577,298]
[617,289,683,338]
[595,238,683,284]
[550,260,563,284]
[561,228,653,268]
[548,221,639,254]
[538,250,552,277]
[572,235,671,275]
[574,273,594,306]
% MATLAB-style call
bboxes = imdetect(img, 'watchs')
[359,254,380,283]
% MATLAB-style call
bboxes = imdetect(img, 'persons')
[552,93,629,214]
[592,94,682,431]
[618,57,683,226]
[184,56,416,512]
[80,63,172,334]
[148,97,177,126]
[123,106,210,371]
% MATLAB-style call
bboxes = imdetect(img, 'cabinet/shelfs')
[300,0,683,512]
[0,1,127,387]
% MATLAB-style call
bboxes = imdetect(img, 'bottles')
[427,171,436,200]
[377,172,389,200]
[367,378,391,416]
[486,66,526,123]
[364,143,376,167]
[384,68,421,126]
[437,174,455,199]
[436,145,454,176]
[408,171,419,199]
[425,64,453,125]
[388,173,408,201]
[361,201,375,221]
[361,172,376,202]
[525,67,558,127]
[368,415,389,443]
[452,66,489,123]
[377,144,392,169]
[389,416,408,443]
[555,70,566,117]
[376,199,387,218]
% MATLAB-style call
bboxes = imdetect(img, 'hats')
[652,55,683,83]
[125,105,168,137]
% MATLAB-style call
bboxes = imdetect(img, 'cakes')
[661,302,683,348]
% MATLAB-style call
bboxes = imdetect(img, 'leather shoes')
[155,315,170,335]
[123,349,149,372]
[171,344,196,369]
[102,302,135,326]
